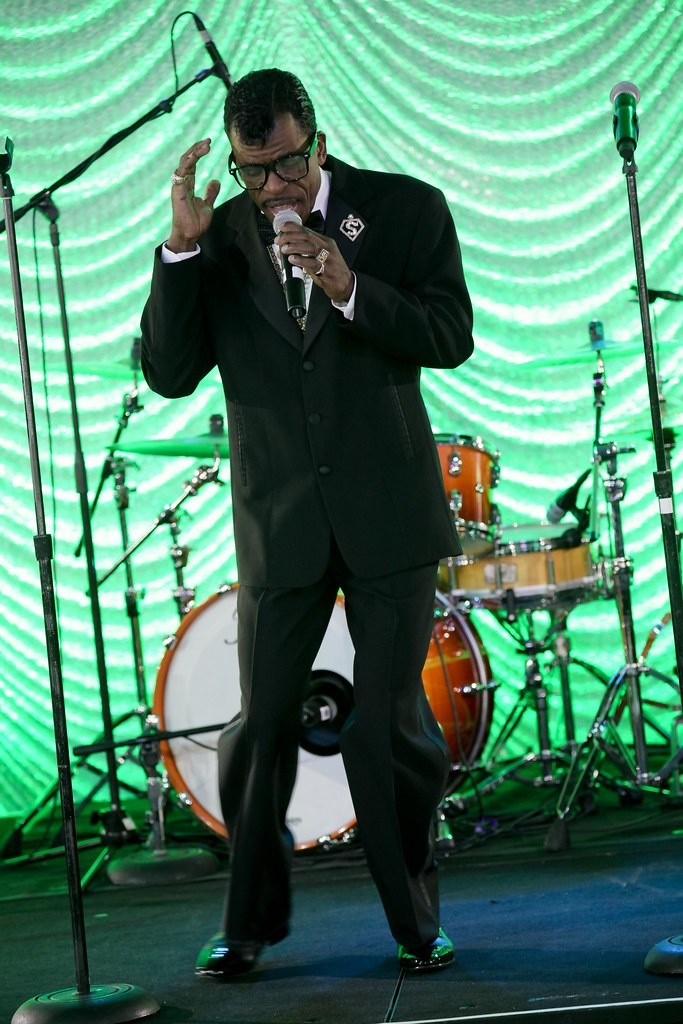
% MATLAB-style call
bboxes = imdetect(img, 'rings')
[170,168,188,184]
[314,262,325,276]
[316,248,330,263]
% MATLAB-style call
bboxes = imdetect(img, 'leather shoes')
[195,932,266,981]
[396,928,456,971]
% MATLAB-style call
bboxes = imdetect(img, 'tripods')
[1,139,683,860]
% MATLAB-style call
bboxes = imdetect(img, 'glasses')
[228,133,318,190]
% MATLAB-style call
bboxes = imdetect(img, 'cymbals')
[106,434,229,459]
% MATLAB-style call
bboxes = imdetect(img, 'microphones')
[589,319,604,343]
[272,210,306,319]
[609,81,640,159]
[194,14,233,91]
[545,467,592,524]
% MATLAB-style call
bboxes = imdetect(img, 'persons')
[140,68,474,980]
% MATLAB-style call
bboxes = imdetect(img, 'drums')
[154,567,498,856]
[439,522,598,604]
[428,431,503,564]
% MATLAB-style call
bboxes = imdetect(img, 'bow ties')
[258,209,325,247]
